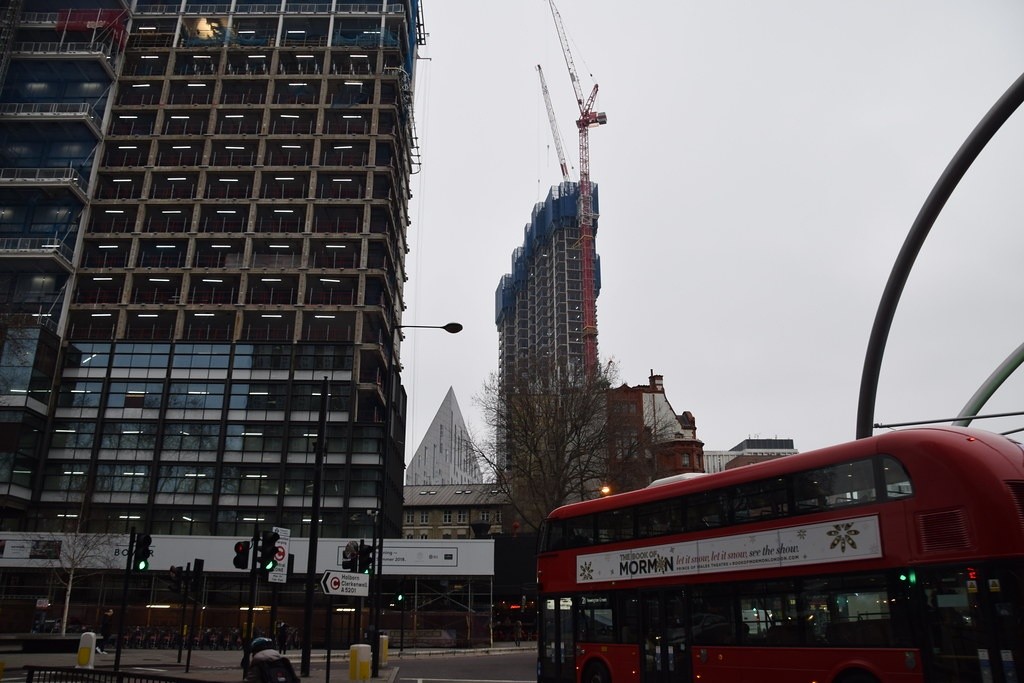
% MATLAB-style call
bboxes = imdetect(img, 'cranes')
[550,0,610,384]
[535,64,569,183]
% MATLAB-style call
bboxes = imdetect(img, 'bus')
[533,423,1024,682]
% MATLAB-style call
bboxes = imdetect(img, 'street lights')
[370,322,464,680]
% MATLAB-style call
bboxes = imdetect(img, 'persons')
[243,635,302,683]
[94,606,114,655]
[278,622,289,654]
[513,617,522,648]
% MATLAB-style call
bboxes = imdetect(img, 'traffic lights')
[169,565,183,594]
[232,540,250,570]
[260,530,279,575]
[342,542,358,574]
[134,532,153,573]
[359,540,374,578]
[395,593,405,604]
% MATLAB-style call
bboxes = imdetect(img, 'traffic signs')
[321,570,369,597]
